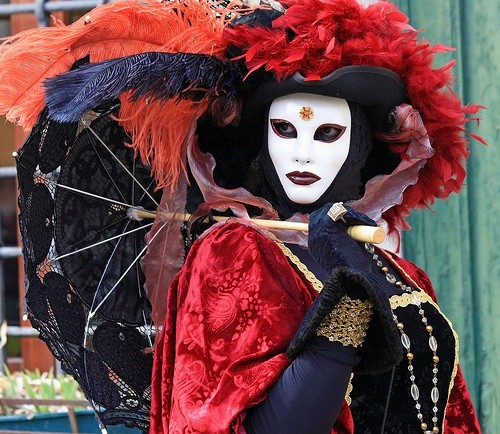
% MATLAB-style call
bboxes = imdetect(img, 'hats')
[188,0,470,245]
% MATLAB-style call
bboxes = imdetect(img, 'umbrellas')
[14,103,384,434]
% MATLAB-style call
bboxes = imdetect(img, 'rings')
[327,204,347,222]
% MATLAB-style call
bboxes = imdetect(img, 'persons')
[149,0,482,434]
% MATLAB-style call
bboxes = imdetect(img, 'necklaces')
[365,241,440,433]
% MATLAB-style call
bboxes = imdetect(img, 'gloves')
[286,202,394,367]
